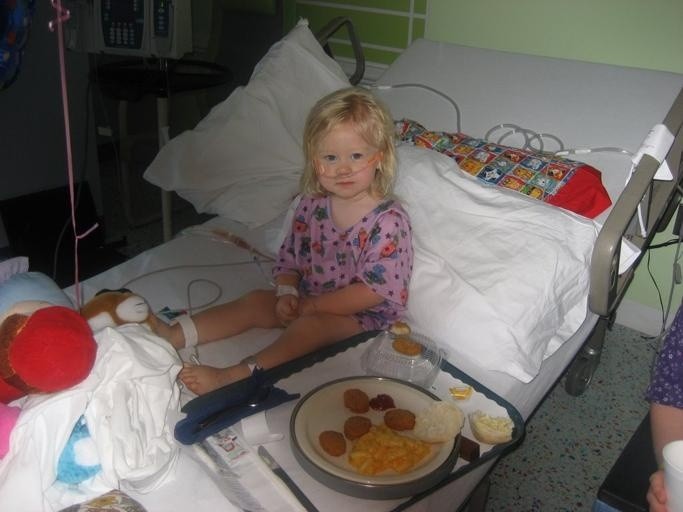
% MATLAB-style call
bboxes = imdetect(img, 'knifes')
[256,444,319,512]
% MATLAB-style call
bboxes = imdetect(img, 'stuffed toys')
[0,288,148,404]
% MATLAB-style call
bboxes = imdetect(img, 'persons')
[147,85,414,397]
[646,295,683,511]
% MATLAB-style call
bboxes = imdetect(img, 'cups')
[660,440,683,512]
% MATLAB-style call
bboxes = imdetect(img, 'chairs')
[1,181,131,289]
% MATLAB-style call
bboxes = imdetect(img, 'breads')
[414,401,465,443]
[468,409,515,445]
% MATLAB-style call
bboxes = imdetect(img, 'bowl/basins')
[358,331,447,389]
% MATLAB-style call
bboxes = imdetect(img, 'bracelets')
[275,284,299,298]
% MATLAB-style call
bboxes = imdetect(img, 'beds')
[65,17,683,510]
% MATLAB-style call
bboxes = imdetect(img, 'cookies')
[319,389,416,456]
[390,324,411,336]
[392,341,424,355]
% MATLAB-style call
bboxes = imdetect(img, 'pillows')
[142,17,641,385]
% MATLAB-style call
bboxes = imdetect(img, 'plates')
[288,376,463,491]
